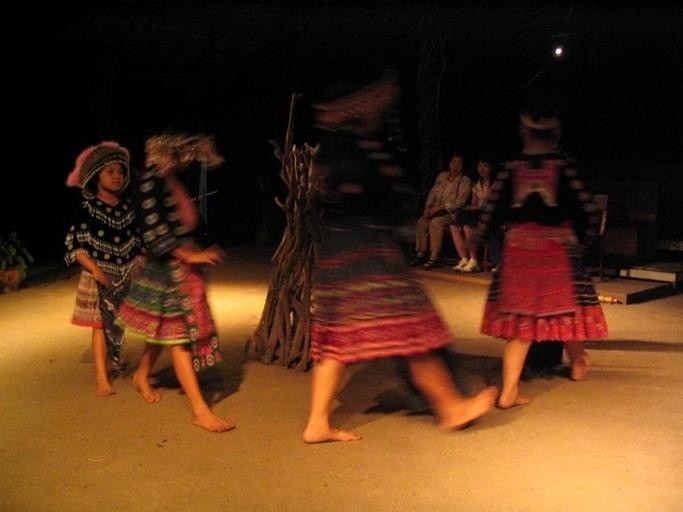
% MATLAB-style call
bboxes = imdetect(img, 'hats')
[144,129,222,177]
[518,113,559,132]
[313,66,402,127]
[65,141,132,200]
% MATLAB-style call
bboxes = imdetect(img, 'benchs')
[477,195,606,273]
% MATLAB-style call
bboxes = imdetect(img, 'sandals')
[422,259,440,269]
[408,257,424,267]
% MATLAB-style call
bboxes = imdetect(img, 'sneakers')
[462,260,481,272]
[452,260,468,273]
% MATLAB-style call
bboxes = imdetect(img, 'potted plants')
[0,241,33,291]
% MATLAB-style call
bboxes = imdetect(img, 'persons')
[111,122,237,433]
[479,114,610,410]
[64,143,147,400]
[447,155,498,274]
[410,152,473,271]
[300,87,501,444]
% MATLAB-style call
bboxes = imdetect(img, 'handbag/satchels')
[454,209,481,229]
